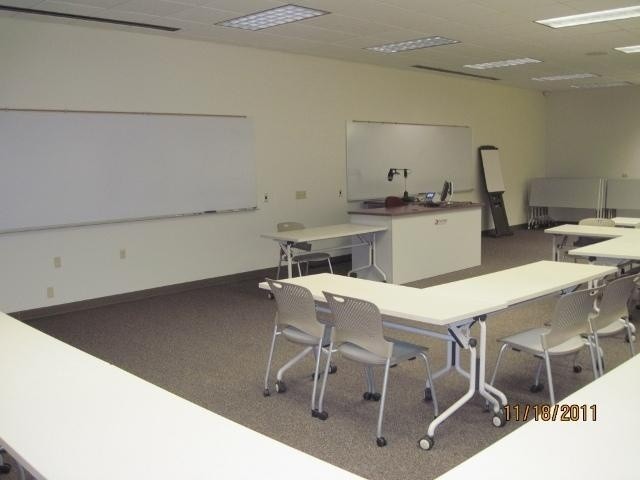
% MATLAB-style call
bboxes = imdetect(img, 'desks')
[260,224,389,281]
[258,259,619,450]
[434,354,639,477]
[544,215,640,265]
[1,311,362,479]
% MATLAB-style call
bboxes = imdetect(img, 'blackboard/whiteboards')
[345,120,475,205]
[1,108,260,235]
[481,147,507,192]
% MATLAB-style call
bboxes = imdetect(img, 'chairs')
[263,276,436,449]
[277,222,333,279]
[481,272,640,413]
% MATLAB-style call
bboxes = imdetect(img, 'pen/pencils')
[205,211,217,213]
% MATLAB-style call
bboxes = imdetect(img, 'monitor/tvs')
[426,192,435,198]
[440,181,449,202]
